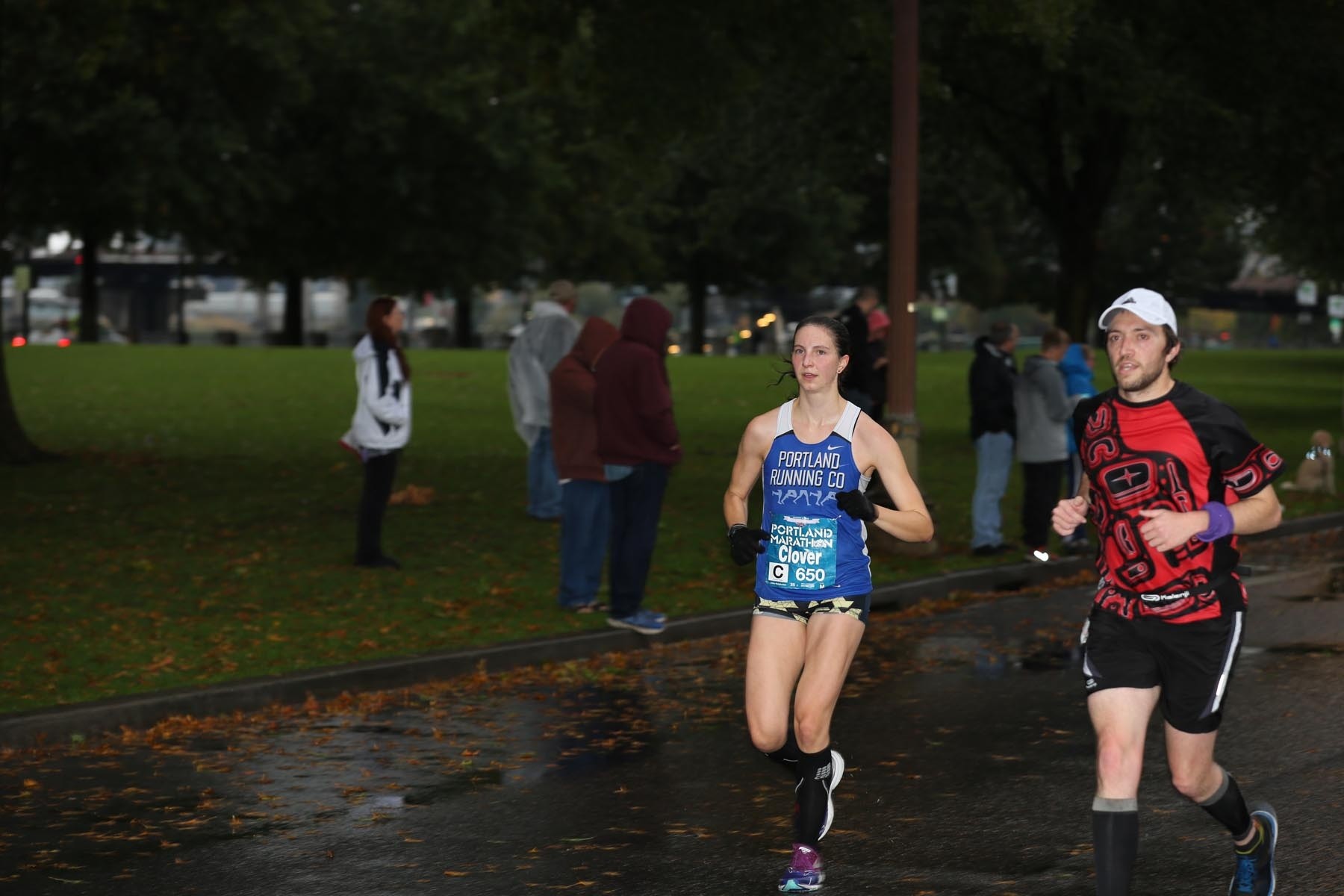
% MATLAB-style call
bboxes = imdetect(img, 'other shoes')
[1060,535,1094,556]
[572,600,610,614]
[972,542,1011,557]
[608,609,668,633]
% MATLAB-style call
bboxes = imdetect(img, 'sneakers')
[1227,810,1278,895]
[777,843,826,892]
[793,751,845,841]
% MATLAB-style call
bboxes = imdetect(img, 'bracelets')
[1196,502,1235,540]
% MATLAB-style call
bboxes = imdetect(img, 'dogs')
[1280,430,1336,495]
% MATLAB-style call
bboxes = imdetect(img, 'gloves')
[835,489,880,524]
[727,523,771,565]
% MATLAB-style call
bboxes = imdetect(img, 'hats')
[1097,288,1178,338]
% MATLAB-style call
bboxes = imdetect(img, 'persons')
[547,316,618,614]
[594,298,681,634]
[519,280,584,521]
[829,291,1099,556]
[349,295,414,568]
[722,315,934,894]
[1050,287,1284,895]
